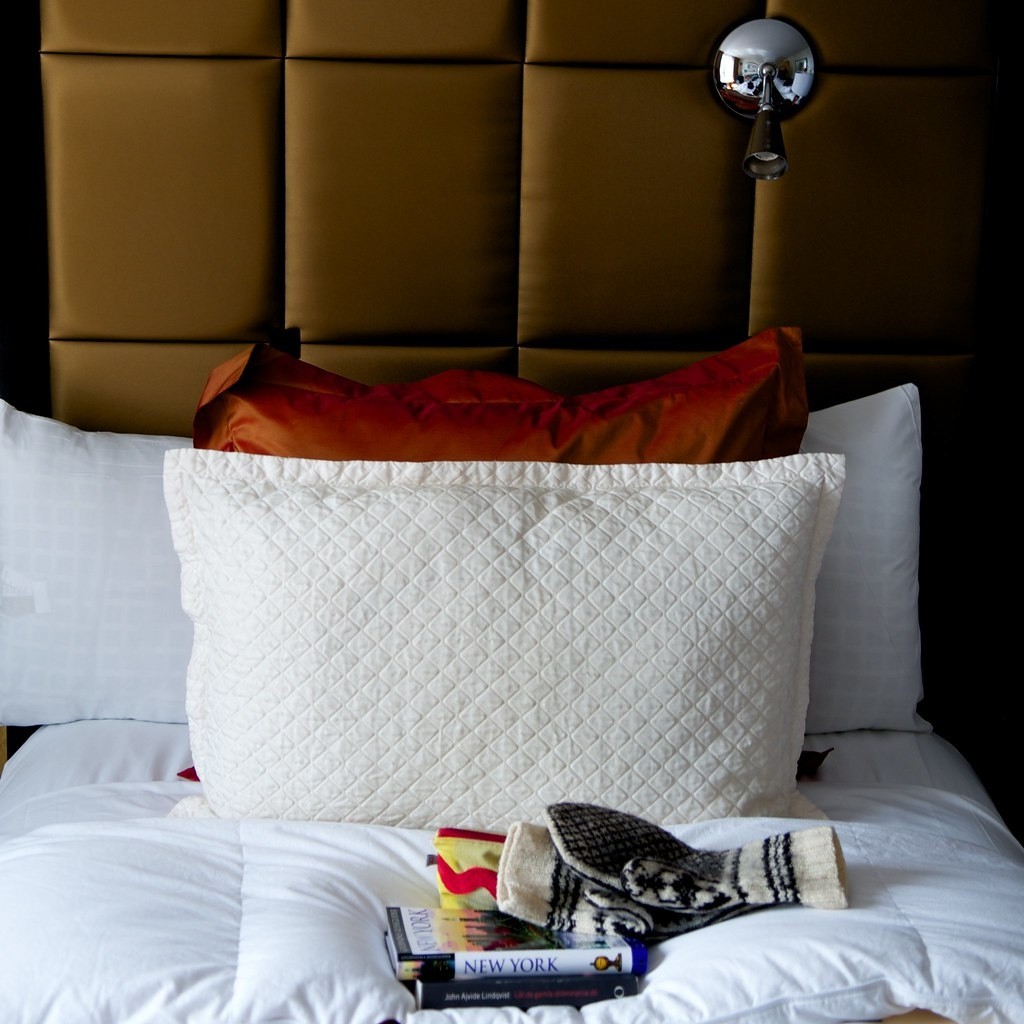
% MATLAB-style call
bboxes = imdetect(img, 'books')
[384,905,648,1011]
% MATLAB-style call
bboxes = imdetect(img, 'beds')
[0,712,1024,1024]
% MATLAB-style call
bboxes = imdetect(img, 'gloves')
[542,802,852,908]
[496,822,777,940]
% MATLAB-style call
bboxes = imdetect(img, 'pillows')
[0,398,191,726]
[198,325,812,483]
[163,447,834,838]
[805,382,933,738]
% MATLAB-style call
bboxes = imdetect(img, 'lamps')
[710,13,820,185]
